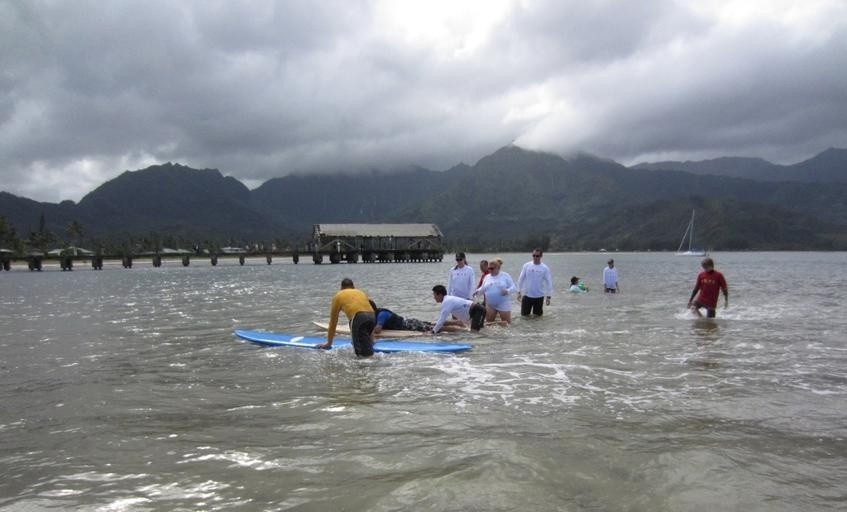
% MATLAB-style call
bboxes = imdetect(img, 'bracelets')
[430,330,434,334]
[546,296,551,299]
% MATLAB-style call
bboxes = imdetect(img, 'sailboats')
[673,207,706,257]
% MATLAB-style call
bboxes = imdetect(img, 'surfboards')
[311,320,470,337]
[235,330,472,353]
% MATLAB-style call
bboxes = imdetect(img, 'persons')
[424,285,509,338]
[516,248,552,315]
[568,276,590,294]
[687,257,729,318]
[447,251,475,318]
[368,299,469,334]
[315,277,378,359]
[475,260,491,305]
[472,258,515,324]
[603,258,620,294]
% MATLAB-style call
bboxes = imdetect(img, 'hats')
[455,252,465,258]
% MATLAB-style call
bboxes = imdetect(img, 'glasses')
[456,259,462,260]
[532,255,540,257]
